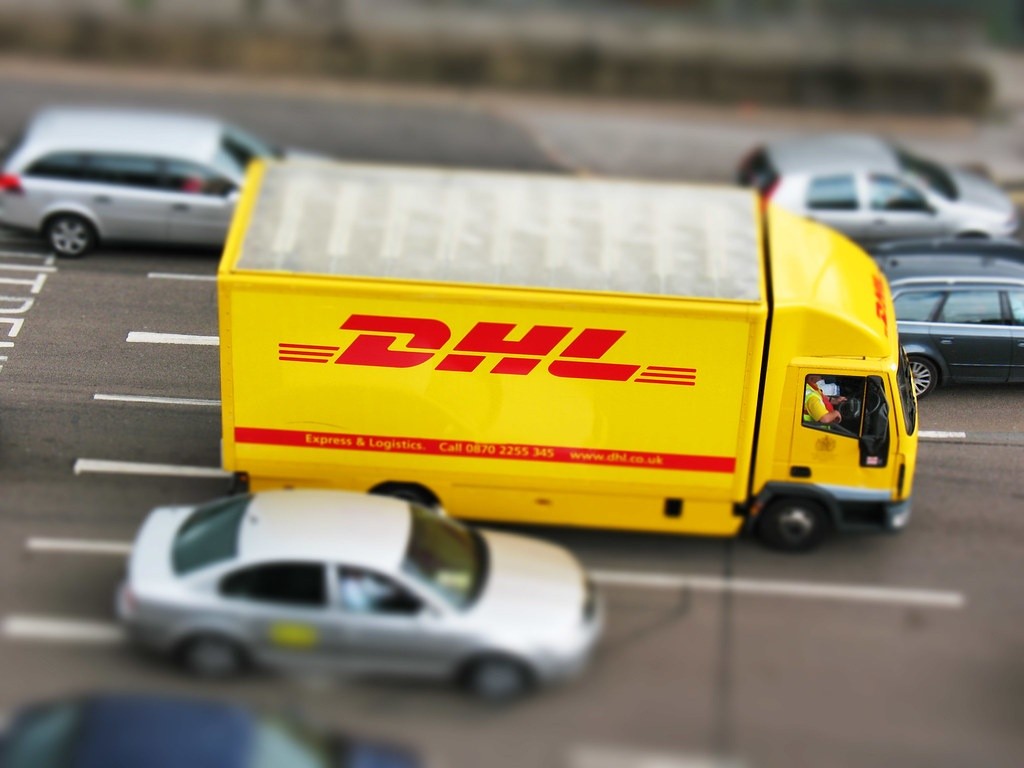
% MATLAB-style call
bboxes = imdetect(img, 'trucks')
[216,146,919,553]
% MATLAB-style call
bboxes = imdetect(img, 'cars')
[0,689,422,768]
[1,102,275,260]
[860,239,1024,402]
[730,128,1019,240]
[113,486,608,707]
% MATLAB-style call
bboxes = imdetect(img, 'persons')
[804,374,847,425]
[344,568,394,613]
